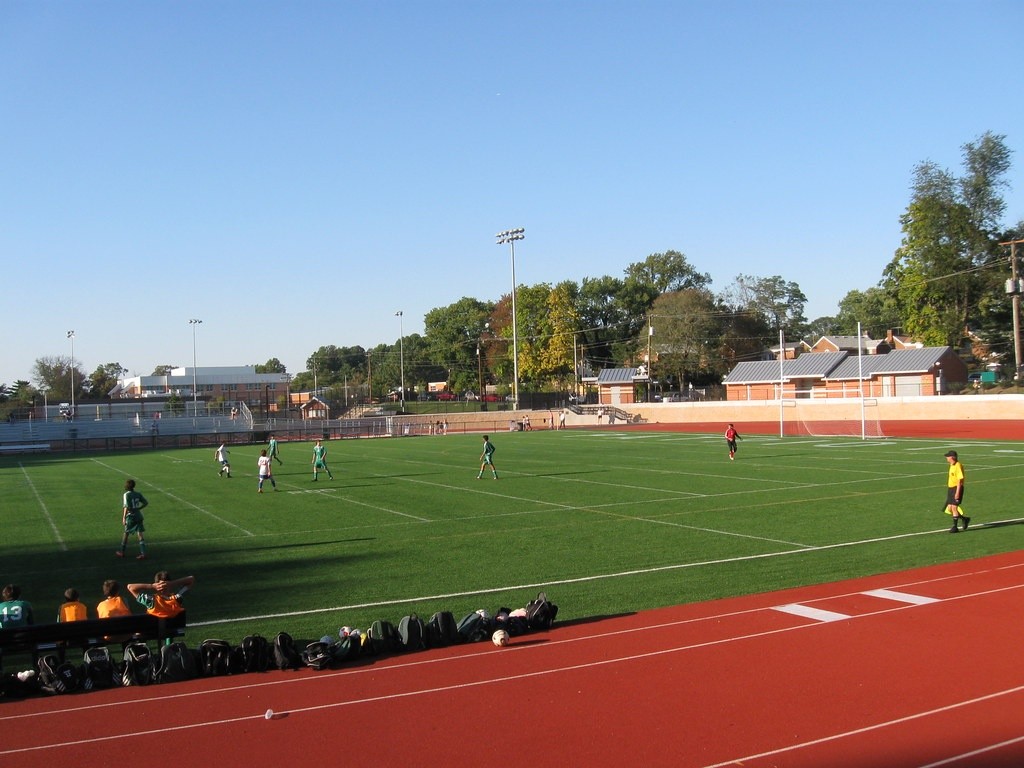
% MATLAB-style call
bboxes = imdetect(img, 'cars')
[417,392,520,404]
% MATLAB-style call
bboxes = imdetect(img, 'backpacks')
[0,592,558,703]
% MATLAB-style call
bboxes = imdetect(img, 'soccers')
[496,612,509,622]
[492,630,511,648]
[338,626,362,639]
[320,636,335,644]
[475,609,489,622]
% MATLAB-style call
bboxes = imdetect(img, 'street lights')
[494,228,525,411]
[66,330,76,416]
[393,311,405,407]
[187,319,204,418]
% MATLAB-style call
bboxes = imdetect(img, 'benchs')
[0,608,186,659]
[0,408,253,456]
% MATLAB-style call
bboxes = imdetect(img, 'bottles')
[264,709,273,719]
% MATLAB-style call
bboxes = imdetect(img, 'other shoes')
[330,476,333,480]
[116,550,125,558]
[950,526,959,533]
[494,476,499,480]
[729,454,734,460]
[962,517,970,529]
[280,462,282,466]
[136,555,146,560]
[477,476,482,479]
[273,487,279,491]
[312,479,317,482]
[258,489,263,493]
[226,475,231,478]
[218,472,222,477]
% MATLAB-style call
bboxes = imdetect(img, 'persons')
[0,584,40,669]
[128,570,196,645]
[475,435,498,480]
[429,419,449,435]
[95,579,133,641]
[311,439,334,481]
[53,587,91,661]
[549,410,566,430]
[115,480,149,560]
[944,451,971,534]
[66,411,73,423]
[151,420,159,435]
[597,407,604,427]
[230,406,238,420]
[266,435,282,465]
[523,415,532,431]
[214,442,233,478]
[258,450,280,492]
[725,424,743,460]
